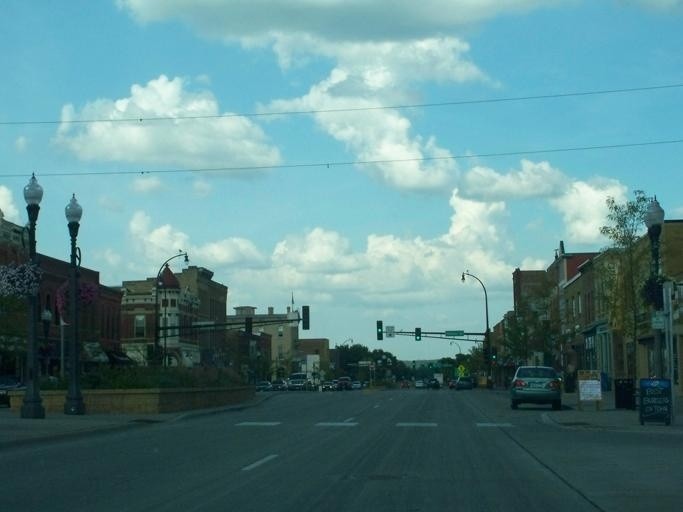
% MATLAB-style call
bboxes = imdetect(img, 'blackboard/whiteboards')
[577,370,601,401]
[639,378,671,422]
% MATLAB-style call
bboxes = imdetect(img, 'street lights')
[152,250,190,367]
[64,191,86,415]
[21,172,46,418]
[39,306,52,381]
[643,195,664,380]
[461,272,492,389]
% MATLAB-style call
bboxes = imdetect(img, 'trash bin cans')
[615,379,634,410]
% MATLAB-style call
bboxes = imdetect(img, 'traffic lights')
[415,328,421,341]
[490,352,497,367]
[377,321,383,340]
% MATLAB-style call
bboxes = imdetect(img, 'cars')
[0,375,28,408]
[255,372,370,392]
[400,377,473,391]
[509,365,563,412]
